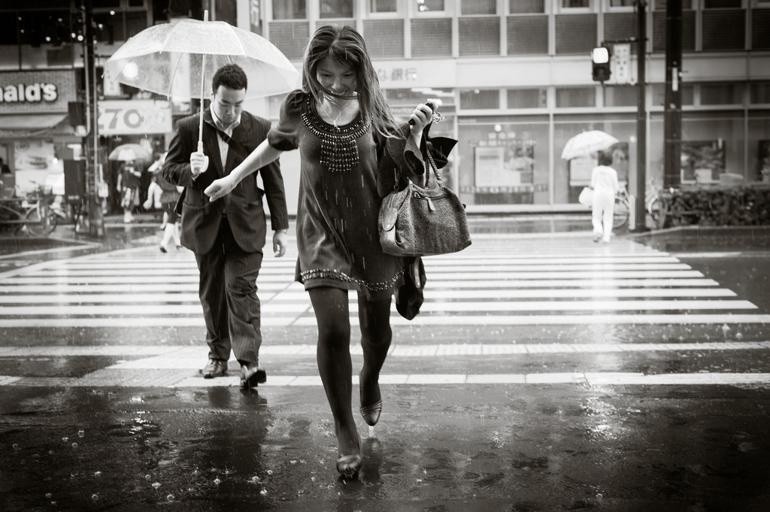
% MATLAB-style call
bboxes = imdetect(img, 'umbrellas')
[99,8,300,156]
[561,127,620,163]
[108,143,149,166]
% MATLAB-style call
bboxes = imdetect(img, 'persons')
[201,22,442,486]
[588,153,620,244]
[116,160,142,223]
[159,61,290,391]
[153,151,185,252]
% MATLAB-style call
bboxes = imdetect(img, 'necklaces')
[324,107,346,132]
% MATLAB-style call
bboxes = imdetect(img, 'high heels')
[376,178,472,258]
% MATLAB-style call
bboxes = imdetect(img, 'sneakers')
[593,233,610,244]
[160,247,167,253]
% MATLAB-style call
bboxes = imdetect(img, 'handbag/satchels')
[578,187,595,209]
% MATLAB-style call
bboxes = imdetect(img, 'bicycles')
[0,187,58,238]
[613,178,674,230]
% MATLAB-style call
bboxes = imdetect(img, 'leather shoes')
[202,359,228,378]
[241,361,266,390]
[336,431,363,479]
[360,398,382,425]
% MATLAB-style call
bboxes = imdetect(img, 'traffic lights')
[590,45,611,82]
